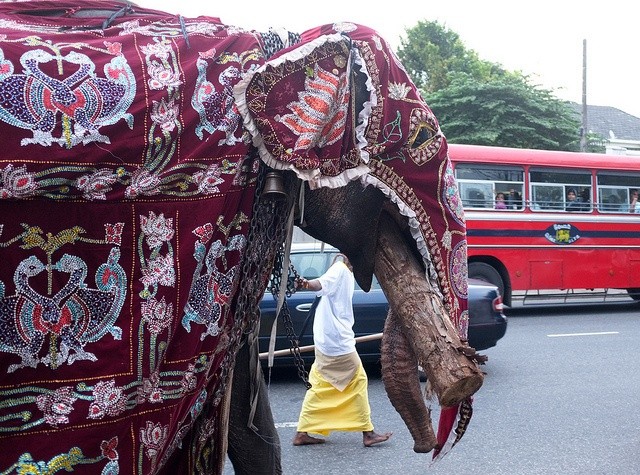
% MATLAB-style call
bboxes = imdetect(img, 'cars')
[260,242,508,382]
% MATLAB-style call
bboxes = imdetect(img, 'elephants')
[0,0,442,475]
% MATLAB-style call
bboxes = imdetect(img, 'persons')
[618,189,640,213]
[566,188,580,211]
[293,256,393,447]
[495,193,507,209]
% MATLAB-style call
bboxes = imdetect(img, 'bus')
[447,141,640,309]
